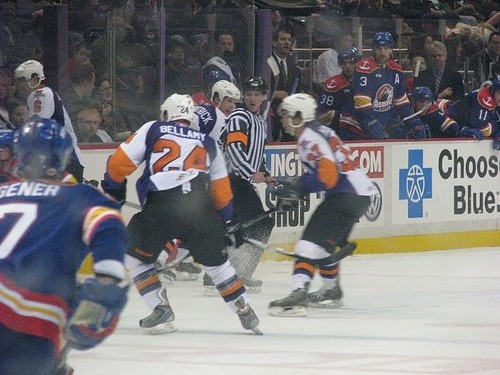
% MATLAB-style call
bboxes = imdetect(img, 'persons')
[0,118,130,375]
[0,0,500,334]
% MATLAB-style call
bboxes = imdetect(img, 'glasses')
[341,60,354,65]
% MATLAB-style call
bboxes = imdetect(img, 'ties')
[434,74,441,98]
[280,60,287,91]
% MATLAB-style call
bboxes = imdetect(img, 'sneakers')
[203,273,263,296]
[267,282,343,317]
[157,263,201,287]
[233,296,263,336]
[139,288,179,335]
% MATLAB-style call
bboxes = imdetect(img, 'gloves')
[65,279,129,352]
[100,173,128,207]
[225,218,243,250]
[268,183,303,202]
[460,128,484,141]
[493,136,500,150]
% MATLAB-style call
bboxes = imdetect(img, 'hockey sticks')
[240,236,357,268]
[123,201,143,210]
[157,207,276,272]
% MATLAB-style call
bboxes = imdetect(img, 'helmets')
[242,76,269,95]
[278,93,318,127]
[210,80,240,108]
[13,60,45,89]
[206,69,231,86]
[372,32,394,47]
[0,129,14,150]
[490,74,500,92]
[338,47,361,67]
[411,85,435,103]
[10,117,73,178]
[160,92,194,129]
